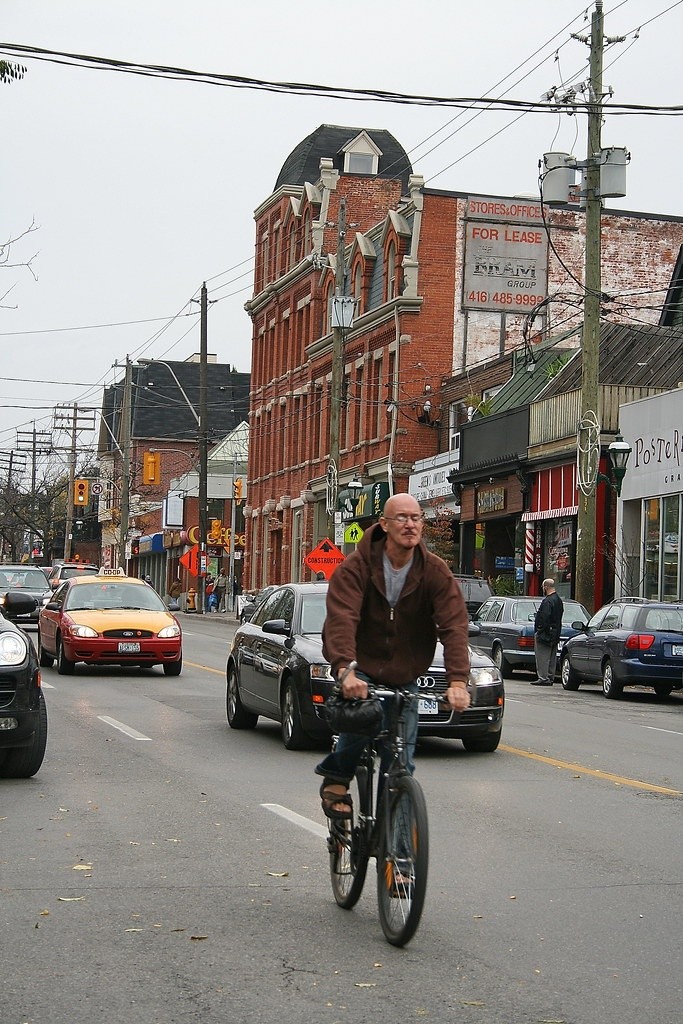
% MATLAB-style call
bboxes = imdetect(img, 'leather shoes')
[530,679,552,686]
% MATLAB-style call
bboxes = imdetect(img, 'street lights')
[137,357,208,615]
[338,473,365,550]
[601,430,633,608]
[78,408,132,572]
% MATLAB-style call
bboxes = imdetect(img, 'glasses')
[385,516,423,522]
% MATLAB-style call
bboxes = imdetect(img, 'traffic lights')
[233,480,242,505]
[74,480,88,505]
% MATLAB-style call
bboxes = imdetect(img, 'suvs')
[0,562,61,624]
[47,562,99,593]
[451,574,497,623]
[559,596,683,699]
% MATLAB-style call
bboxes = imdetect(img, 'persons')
[205,568,238,613]
[169,577,182,604]
[530,579,564,687]
[315,493,470,899]
[141,573,153,587]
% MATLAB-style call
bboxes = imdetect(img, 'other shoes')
[221,609,226,613]
[214,609,217,613]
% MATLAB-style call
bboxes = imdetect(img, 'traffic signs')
[304,538,347,581]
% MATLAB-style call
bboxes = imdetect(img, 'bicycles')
[327,685,473,949]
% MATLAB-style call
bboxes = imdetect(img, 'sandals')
[320,777,353,820]
[392,871,413,899]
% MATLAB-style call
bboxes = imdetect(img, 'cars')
[225,580,505,752]
[38,568,183,677]
[464,595,593,679]
[6,566,53,587]
[0,591,47,781]
[239,584,280,626]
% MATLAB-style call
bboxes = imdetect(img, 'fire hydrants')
[185,587,196,610]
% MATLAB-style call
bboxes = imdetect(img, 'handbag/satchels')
[209,593,217,607]
[538,624,561,647]
[206,585,214,595]
